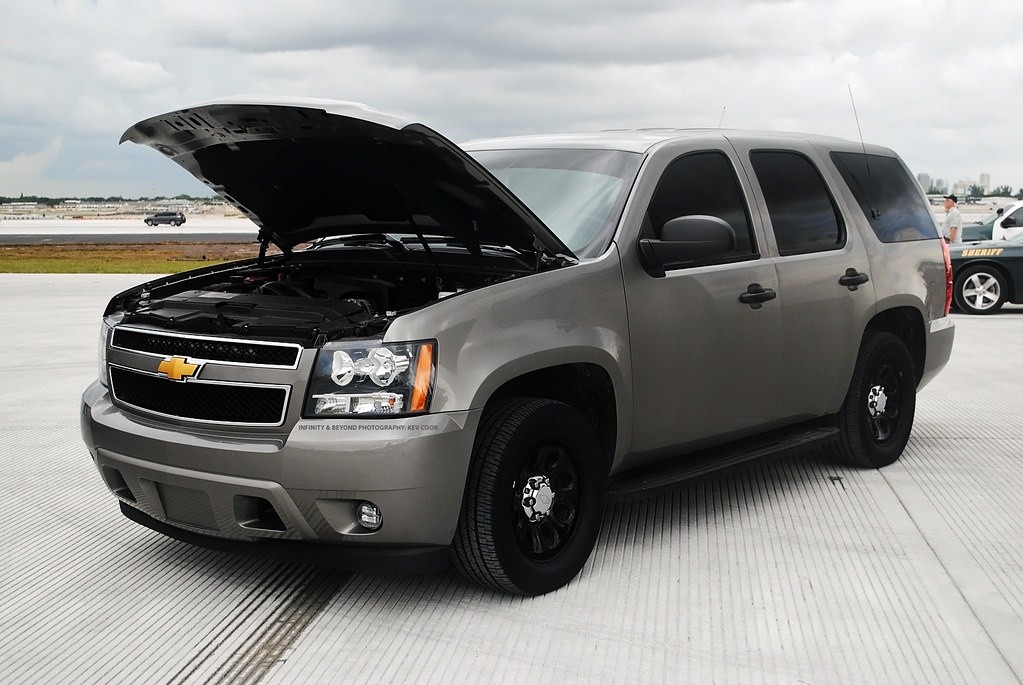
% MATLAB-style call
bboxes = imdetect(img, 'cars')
[927,192,1023,315]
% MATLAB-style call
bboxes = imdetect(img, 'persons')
[940,195,963,243]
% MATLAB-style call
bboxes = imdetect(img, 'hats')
[944,195,957,203]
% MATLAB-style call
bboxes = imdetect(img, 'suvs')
[144,210,186,227]
[79,92,957,600]
